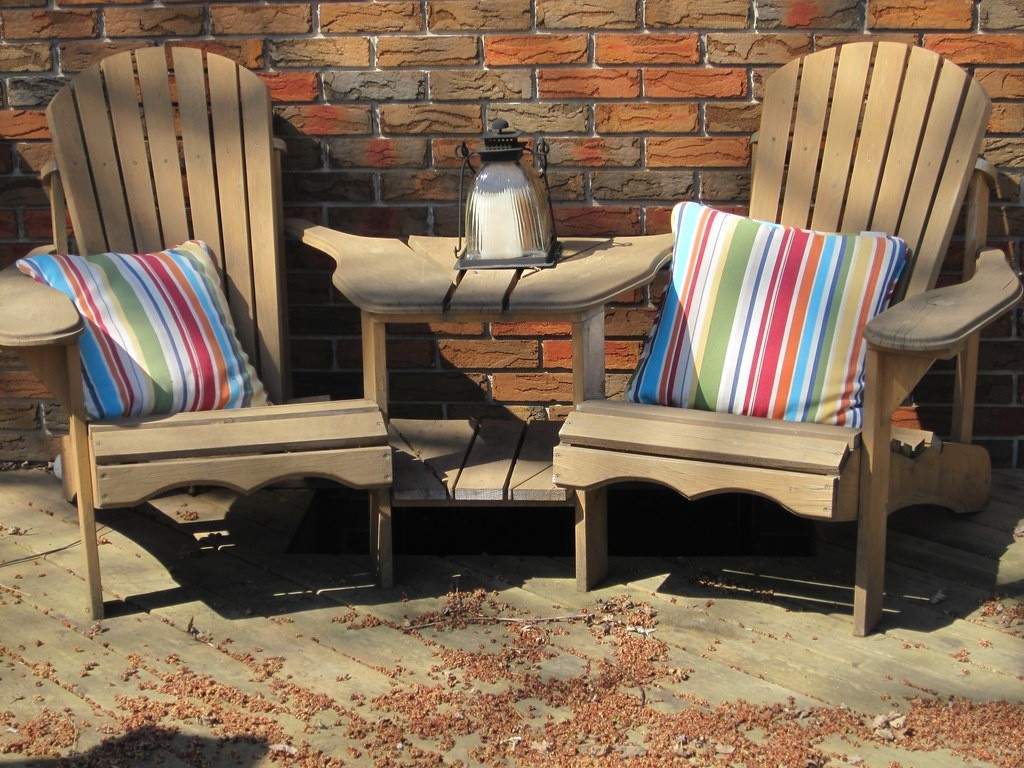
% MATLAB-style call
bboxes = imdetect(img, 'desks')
[284,219,676,595]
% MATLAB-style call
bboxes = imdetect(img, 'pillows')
[625,200,910,428]
[14,239,273,422]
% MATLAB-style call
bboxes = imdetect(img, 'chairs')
[0,47,392,621]
[551,42,1023,635]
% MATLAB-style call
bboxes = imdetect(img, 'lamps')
[454,116,562,270]
